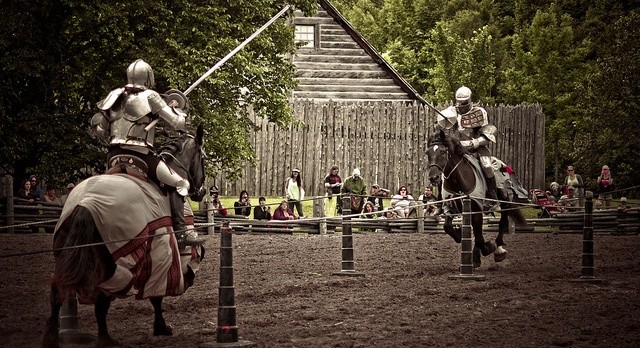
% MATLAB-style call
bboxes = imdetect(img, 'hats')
[292,168,300,173]
[372,184,380,189]
[209,186,219,193]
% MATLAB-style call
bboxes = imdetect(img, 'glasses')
[400,189,406,191]
[568,170,573,172]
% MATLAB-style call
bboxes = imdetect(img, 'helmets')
[454,86,474,115]
[124,58,155,91]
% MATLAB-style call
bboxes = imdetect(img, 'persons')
[594,164,613,210]
[557,186,580,215]
[550,182,561,201]
[374,209,402,234]
[28,175,44,201]
[618,196,632,211]
[438,86,499,206]
[357,201,380,234]
[59,183,75,207]
[323,166,342,216]
[545,190,556,202]
[340,169,367,214]
[233,190,251,233]
[367,184,389,217]
[41,185,62,234]
[283,169,305,219]
[201,186,228,234]
[252,197,270,235]
[90,59,207,248]
[564,166,584,206]
[390,187,416,219]
[268,201,298,234]
[15,181,38,233]
[418,188,441,234]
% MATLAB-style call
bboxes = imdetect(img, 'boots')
[486,175,497,199]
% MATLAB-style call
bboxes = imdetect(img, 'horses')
[39,121,208,347]
[423,127,530,268]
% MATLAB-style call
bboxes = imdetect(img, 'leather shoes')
[181,233,210,248]
